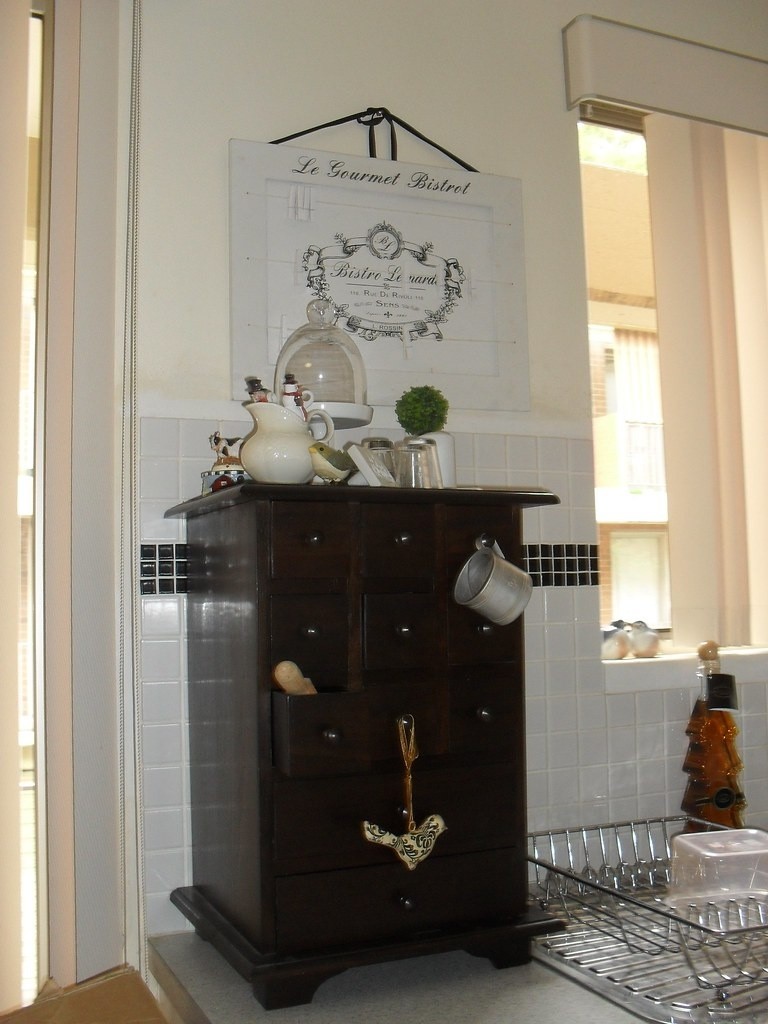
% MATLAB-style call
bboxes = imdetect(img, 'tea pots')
[239,398,334,485]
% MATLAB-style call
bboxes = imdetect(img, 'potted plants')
[395,385,458,489]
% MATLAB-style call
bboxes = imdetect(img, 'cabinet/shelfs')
[164,483,565,1013]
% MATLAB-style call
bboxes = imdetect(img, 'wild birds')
[308,442,358,485]
[603,620,660,659]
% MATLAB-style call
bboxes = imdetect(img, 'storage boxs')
[664,827,768,930]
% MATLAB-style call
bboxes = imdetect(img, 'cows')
[209,432,244,459]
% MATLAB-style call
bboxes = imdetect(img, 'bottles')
[247,379,278,406]
[282,373,308,422]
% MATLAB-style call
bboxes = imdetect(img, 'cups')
[453,531,532,626]
[402,437,444,491]
[368,440,398,481]
[396,444,431,489]
